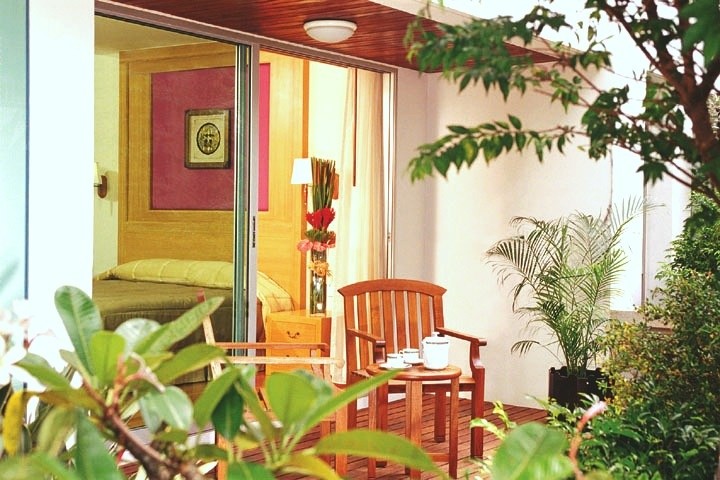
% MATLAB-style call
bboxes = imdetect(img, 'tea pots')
[422,332,450,370]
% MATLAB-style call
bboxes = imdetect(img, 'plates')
[404,359,424,364]
[380,364,412,370]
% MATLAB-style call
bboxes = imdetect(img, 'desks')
[366,362,462,480]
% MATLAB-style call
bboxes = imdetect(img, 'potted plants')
[486,192,669,427]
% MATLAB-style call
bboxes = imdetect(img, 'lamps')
[304,18,358,43]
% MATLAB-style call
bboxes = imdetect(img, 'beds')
[92,277,294,383]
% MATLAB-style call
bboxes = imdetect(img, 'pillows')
[100,258,291,314]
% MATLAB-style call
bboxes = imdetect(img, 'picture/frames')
[185,108,230,169]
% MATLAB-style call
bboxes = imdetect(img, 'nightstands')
[262,311,329,386]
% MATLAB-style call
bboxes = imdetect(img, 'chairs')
[336,279,489,464]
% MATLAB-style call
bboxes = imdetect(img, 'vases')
[312,276,324,316]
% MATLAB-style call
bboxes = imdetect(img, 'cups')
[387,355,404,366]
[400,349,419,361]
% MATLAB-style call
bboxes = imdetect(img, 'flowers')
[297,158,340,279]
